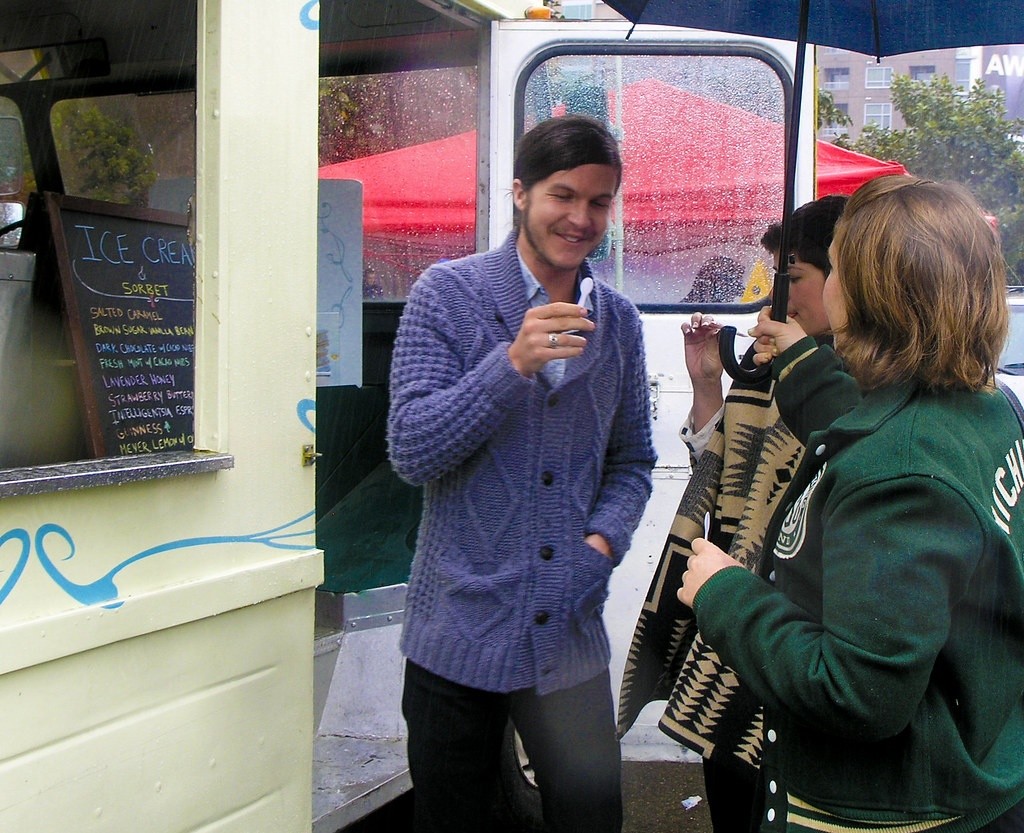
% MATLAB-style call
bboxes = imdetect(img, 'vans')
[0,0,816,833]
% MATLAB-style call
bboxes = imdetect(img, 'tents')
[319,76,909,255]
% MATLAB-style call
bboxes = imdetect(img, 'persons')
[617,176,1023,833]
[384,116,656,833]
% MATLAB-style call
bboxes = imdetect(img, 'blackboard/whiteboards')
[16,194,195,457]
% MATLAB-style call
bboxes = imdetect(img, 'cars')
[990,286,1024,410]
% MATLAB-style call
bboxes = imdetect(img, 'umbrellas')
[602,1,1024,385]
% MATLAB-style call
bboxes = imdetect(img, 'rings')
[548,333,558,349]
